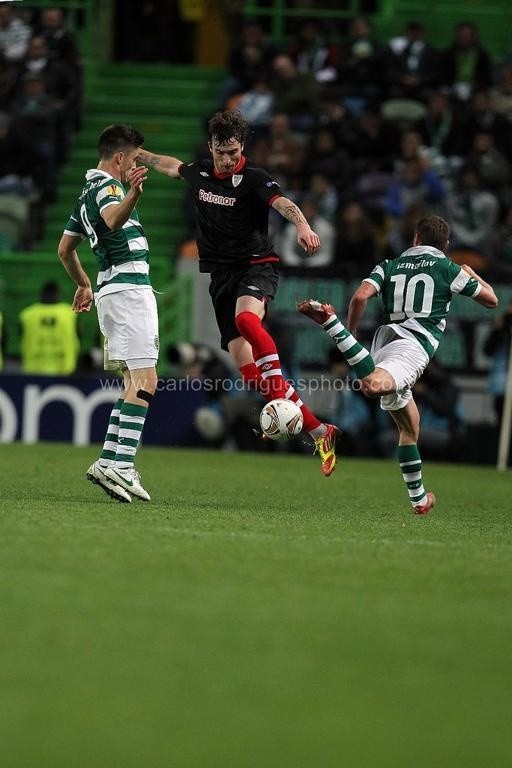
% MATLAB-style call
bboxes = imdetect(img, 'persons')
[132,105,345,479]
[0,310,8,375]
[293,213,502,518]
[56,120,162,508]
[87,321,128,378]
[17,280,83,378]
[0,2,89,253]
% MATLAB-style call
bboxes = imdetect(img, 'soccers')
[259,398,304,442]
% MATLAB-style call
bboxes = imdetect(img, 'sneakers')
[262,433,269,442]
[296,299,336,325]
[314,424,342,477]
[86,460,151,503]
[412,492,435,516]
